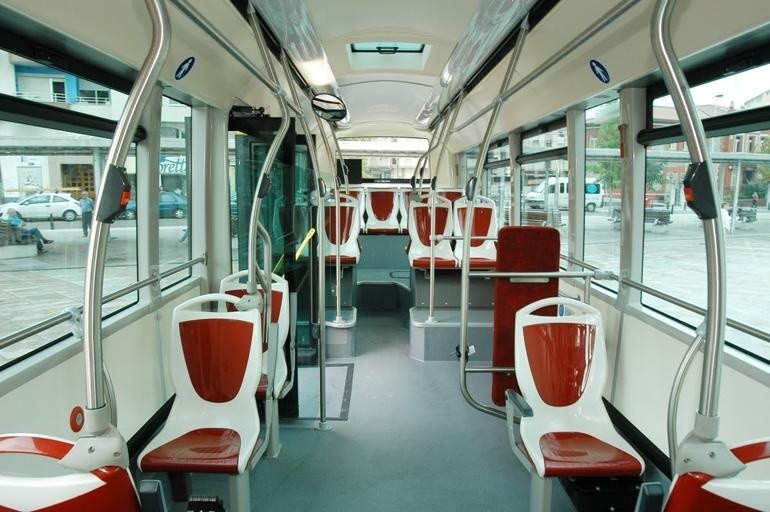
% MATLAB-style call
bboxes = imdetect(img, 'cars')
[115,189,187,219]
[230,190,237,212]
[0,191,83,222]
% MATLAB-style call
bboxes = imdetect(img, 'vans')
[525,176,604,212]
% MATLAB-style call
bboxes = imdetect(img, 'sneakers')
[39,249,47,253]
[43,240,54,244]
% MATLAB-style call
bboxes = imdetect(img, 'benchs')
[610,205,673,226]
[503,206,566,229]
[0,217,36,246]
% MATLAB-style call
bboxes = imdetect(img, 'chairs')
[0,434,137,512]
[135,268,297,511]
[661,439,770,511]
[314,184,500,280]
[506,296,647,511]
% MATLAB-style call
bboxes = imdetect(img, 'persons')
[79,190,94,238]
[6,206,53,251]
[720,203,732,231]
[750,191,759,208]
